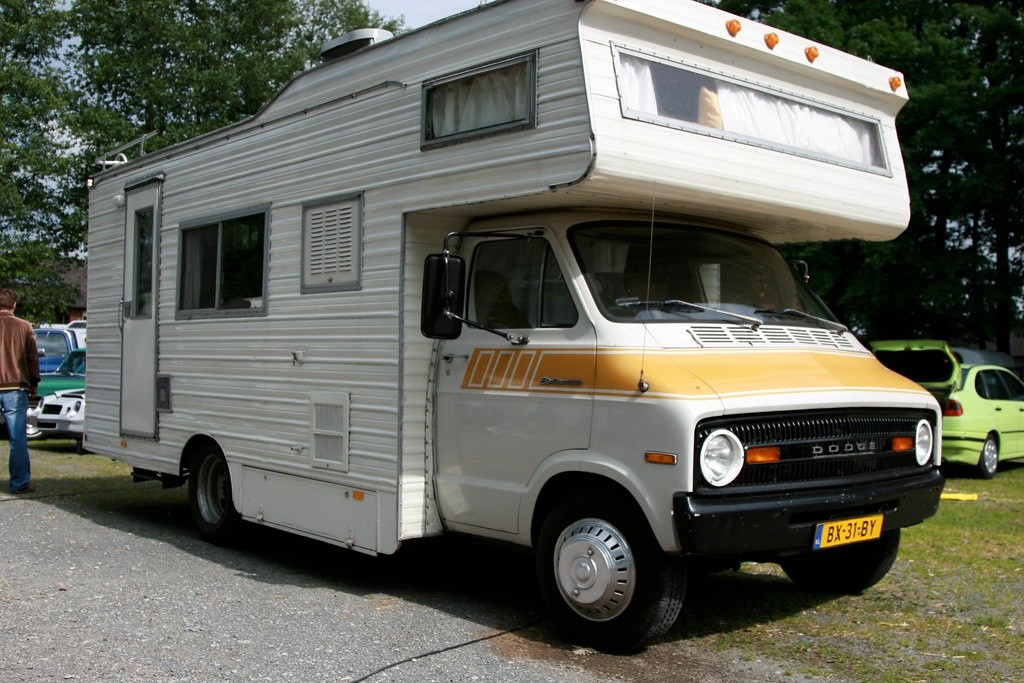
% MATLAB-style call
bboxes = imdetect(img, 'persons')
[739,263,780,312]
[222,237,263,299]
[0,288,41,494]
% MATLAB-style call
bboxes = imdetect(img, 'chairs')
[474,270,533,329]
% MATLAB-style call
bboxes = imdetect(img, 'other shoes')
[11,484,35,494]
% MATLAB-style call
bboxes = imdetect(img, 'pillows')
[699,86,724,130]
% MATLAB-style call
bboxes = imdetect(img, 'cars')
[25,319,87,442]
[872,342,1024,481]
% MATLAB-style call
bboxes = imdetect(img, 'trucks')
[85,0,951,633]
